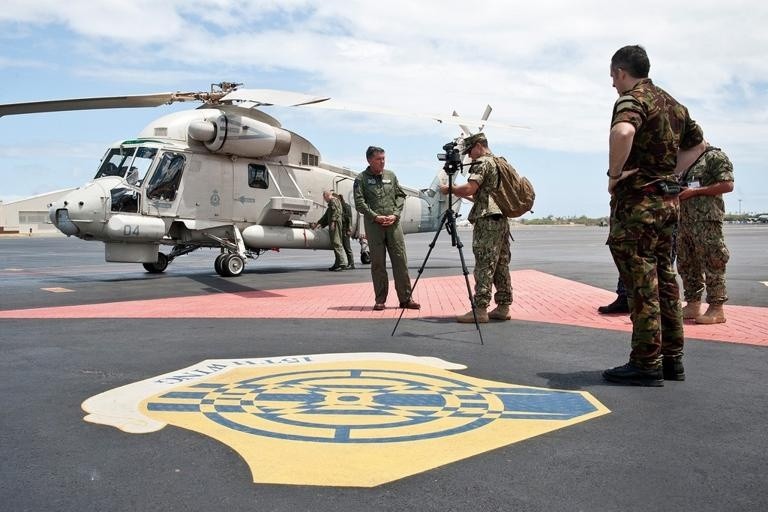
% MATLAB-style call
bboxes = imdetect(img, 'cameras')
[437,143,464,176]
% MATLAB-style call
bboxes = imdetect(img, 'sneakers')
[695,304,726,324]
[456,308,489,323]
[488,307,512,320]
[373,304,385,310]
[400,301,420,309]
[682,301,701,319]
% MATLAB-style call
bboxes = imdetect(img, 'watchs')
[606,169,622,180]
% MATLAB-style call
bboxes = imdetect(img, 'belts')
[642,185,660,193]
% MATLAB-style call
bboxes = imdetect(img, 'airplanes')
[0,82,493,277]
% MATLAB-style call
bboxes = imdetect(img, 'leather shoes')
[662,358,685,381]
[598,296,630,314]
[329,266,339,271]
[334,266,345,271]
[346,264,355,269]
[603,361,664,387]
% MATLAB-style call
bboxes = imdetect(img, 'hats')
[461,133,487,154]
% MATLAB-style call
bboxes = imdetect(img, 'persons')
[354,145,421,310]
[601,44,707,386]
[439,133,535,323]
[677,123,735,324]
[312,190,348,272]
[337,194,355,270]
[597,274,629,314]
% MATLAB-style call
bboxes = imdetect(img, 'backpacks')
[483,153,535,219]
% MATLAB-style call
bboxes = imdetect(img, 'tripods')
[392,176,483,344]
